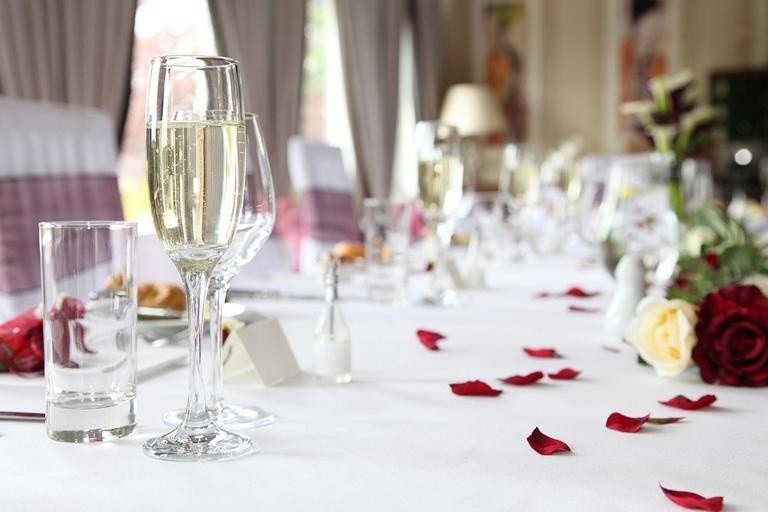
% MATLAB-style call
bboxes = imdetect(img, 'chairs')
[0,94,124,309]
[287,139,364,263]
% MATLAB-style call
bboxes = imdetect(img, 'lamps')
[439,82,510,190]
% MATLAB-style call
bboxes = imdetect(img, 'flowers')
[624,199,768,391]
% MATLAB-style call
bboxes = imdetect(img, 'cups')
[361,196,412,301]
[38,213,135,440]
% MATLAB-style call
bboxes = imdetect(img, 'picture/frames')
[471,3,543,152]
[605,0,682,155]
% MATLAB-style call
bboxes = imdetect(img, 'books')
[0,383,47,421]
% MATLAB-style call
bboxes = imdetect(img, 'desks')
[0,239,768,511]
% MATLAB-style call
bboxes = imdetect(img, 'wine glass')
[404,119,711,304]
[145,51,250,461]
[168,108,269,430]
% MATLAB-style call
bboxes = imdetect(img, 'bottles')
[312,256,349,383]
[601,255,650,355]
